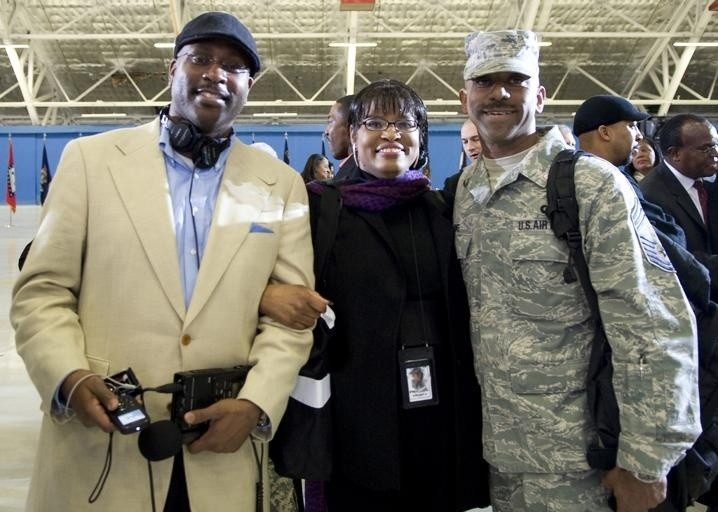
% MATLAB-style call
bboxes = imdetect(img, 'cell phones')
[100,394,151,434]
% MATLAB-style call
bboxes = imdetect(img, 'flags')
[39,132,51,208]
[6,135,16,214]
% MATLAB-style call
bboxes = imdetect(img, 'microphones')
[137,419,211,461]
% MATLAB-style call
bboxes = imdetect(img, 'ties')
[694,181,708,223]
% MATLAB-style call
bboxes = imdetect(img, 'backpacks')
[548,148,717,508]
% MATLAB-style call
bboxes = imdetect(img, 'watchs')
[627,469,662,484]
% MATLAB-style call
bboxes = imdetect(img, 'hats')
[573,95,650,135]
[463,30,539,81]
[175,11,260,73]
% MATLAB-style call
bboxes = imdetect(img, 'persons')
[444,119,484,225]
[9,11,317,510]
[259,74,491,508]
[409,368,426,390]
[444,22,705,511]
[572,95,718,305]
[300,95,355,185]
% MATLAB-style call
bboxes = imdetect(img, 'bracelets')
[258,408,270,429]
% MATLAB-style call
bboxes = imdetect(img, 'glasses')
[355,118,419,133]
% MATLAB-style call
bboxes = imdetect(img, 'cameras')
[103,367,143,397]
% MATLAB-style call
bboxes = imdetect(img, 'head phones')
[159,104,235,169]
